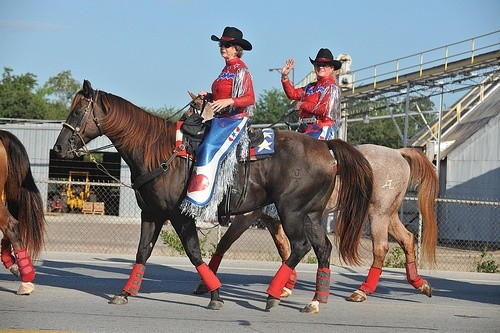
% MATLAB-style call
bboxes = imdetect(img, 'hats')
[211,27,252,50]
[309,48,342,70]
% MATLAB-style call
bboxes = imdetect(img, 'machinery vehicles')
[62,171,91,212]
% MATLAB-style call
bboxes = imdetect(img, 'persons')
[180,26,255,229]
[281,48,342,140]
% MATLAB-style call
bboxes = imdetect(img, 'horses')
[52,79,440,315]
[0,130,48,295]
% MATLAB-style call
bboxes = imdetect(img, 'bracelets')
[228,98,234,108]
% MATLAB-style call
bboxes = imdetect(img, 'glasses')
[315,62,333,67]
[218,42,237,48]
